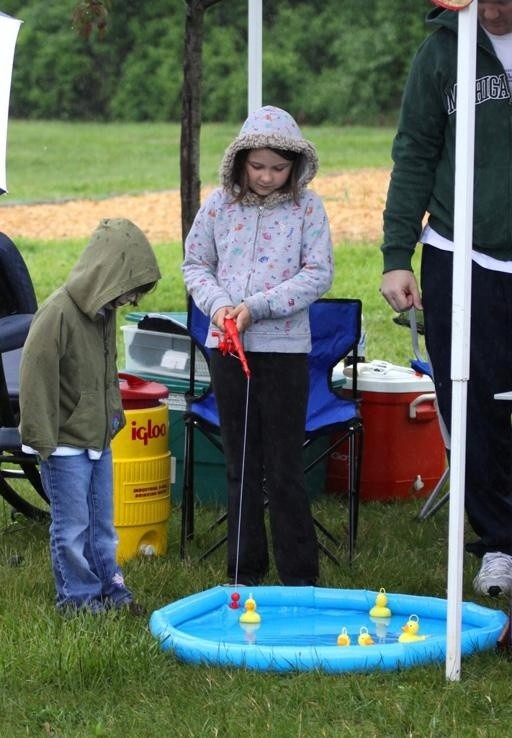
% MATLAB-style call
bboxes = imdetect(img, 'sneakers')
[472,551,512,601]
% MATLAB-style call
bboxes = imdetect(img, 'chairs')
[180,294,363,569]
[0,231,50,523]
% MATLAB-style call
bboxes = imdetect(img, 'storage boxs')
[120,311,344,381]
[126,370,346,509]
[325,359,446,501]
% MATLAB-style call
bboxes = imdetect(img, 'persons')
[181,106,333,585]
[17,217,162,615]
[379,0,512,595]
